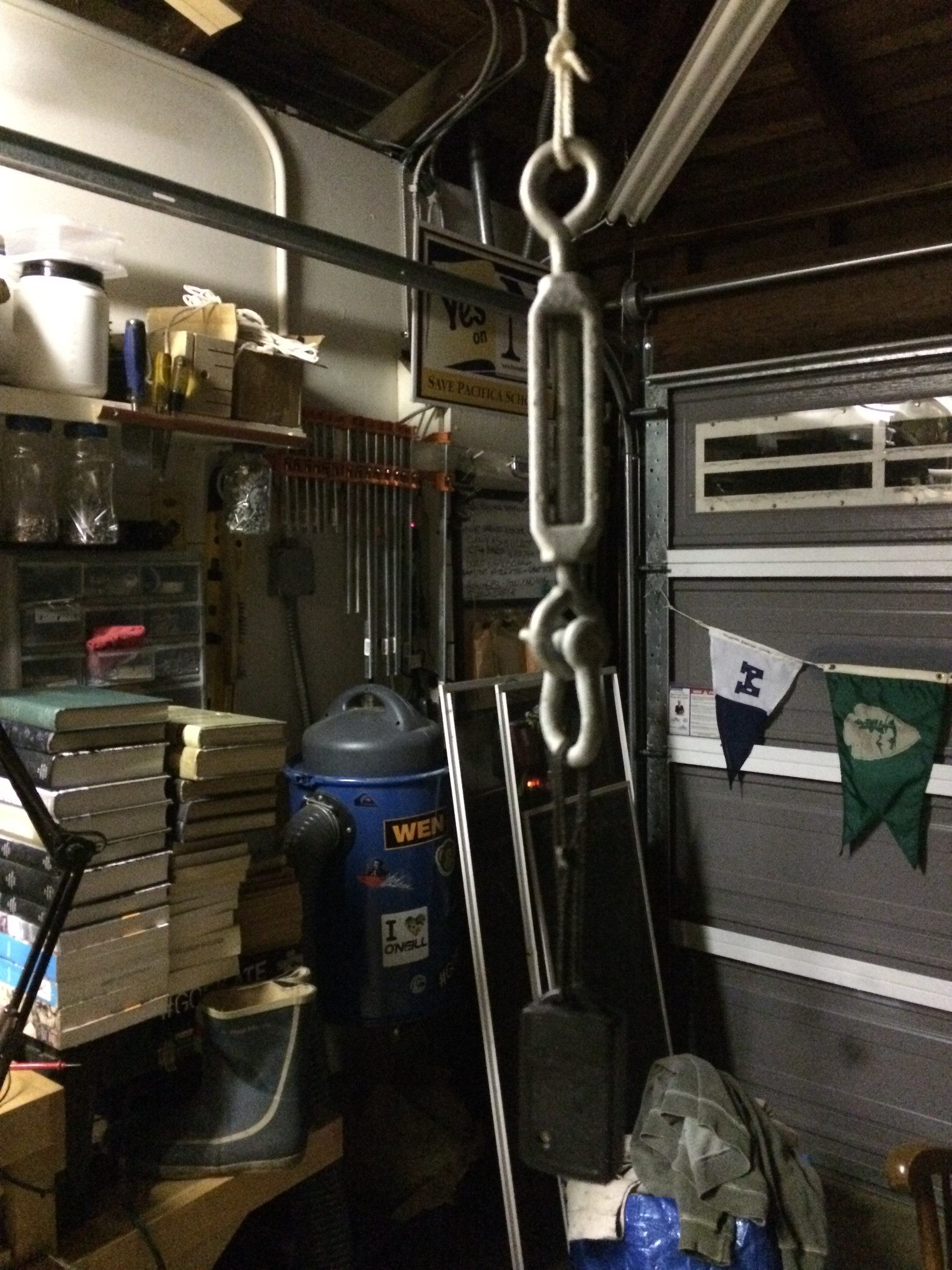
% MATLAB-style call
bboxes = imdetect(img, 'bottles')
[0,415,60,544]
[12,257,111,397]
[63,421,122,539]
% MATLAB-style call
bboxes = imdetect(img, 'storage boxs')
[145,303,326,427]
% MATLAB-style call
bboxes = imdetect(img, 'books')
[0,743,171,792]
[0,904,172,953]
[169,794,293,822]
[172,813,277,842]
[0,720,168,754]
[0,775,171,819]
[0,851,174,905]
[166,770,283,802]
[0,974,179,1032]
[0,828,174,876]
[0,799,174,851]
[164,740,294,780]
[168,830,273,856]
[169,842,304,997]
[0,686,174,732]
[0,994,174,1051]
[0,924,170,981]
[0,950,177,1009]
[166,705,287,750]
[0,884,172,932]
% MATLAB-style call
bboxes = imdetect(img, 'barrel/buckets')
[289,684,473,1024]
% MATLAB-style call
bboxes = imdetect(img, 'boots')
[157,966,316,1179]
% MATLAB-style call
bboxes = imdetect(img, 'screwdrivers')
[161,356,191,474]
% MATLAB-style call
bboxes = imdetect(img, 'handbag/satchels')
[516,993,631,1182]
[571,1183,782,1269]
